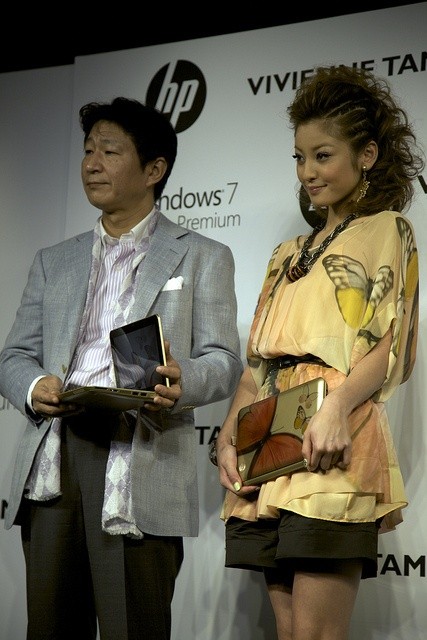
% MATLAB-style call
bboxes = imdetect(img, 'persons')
[215,69,418,640]
[0,96,243,640]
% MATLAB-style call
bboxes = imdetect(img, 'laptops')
[55,314,169,413]
[236,377,329,486]
